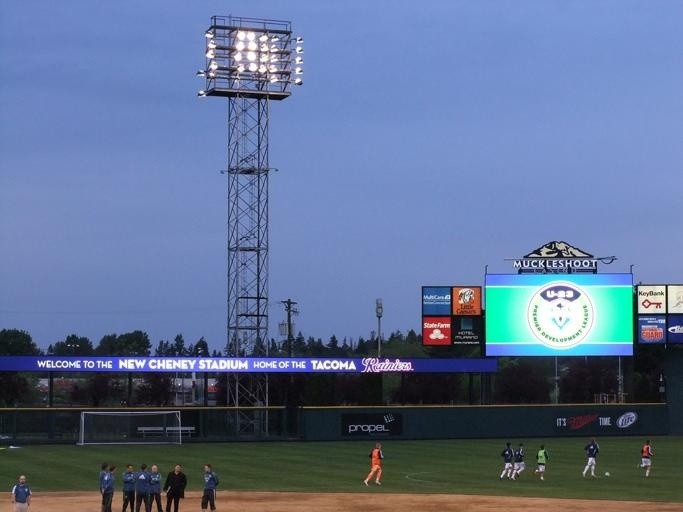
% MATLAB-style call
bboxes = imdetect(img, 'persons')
[534,444,549,481]
[511,443,525,480]
[363,442,384,486]
[582,438,600,478]
[9,475,32,512]
[499,441,514,481]
[636,439,655,476]
[201,464,219,512]
[98,462,187,512]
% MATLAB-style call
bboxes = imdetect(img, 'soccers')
[605,472,610,476]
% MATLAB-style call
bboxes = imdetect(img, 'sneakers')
[500,471,546,481]
[375,480,382,485]
[364,480,368,486]
